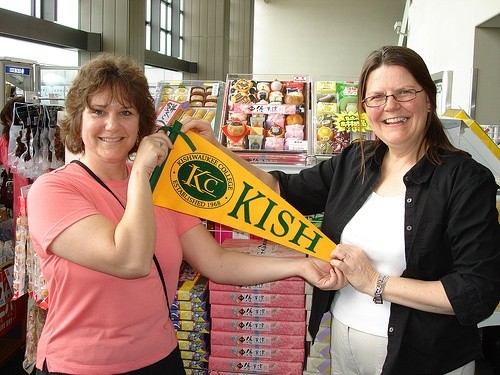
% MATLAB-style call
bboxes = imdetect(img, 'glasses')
[360,87,424,107]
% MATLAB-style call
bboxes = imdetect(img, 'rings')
[342,254,346,259]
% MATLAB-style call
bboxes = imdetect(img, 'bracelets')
[373,274,387,303]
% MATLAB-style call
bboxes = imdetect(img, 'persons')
[179,46,500,375]
[27,55,348,375]
[0,98,24,208]
